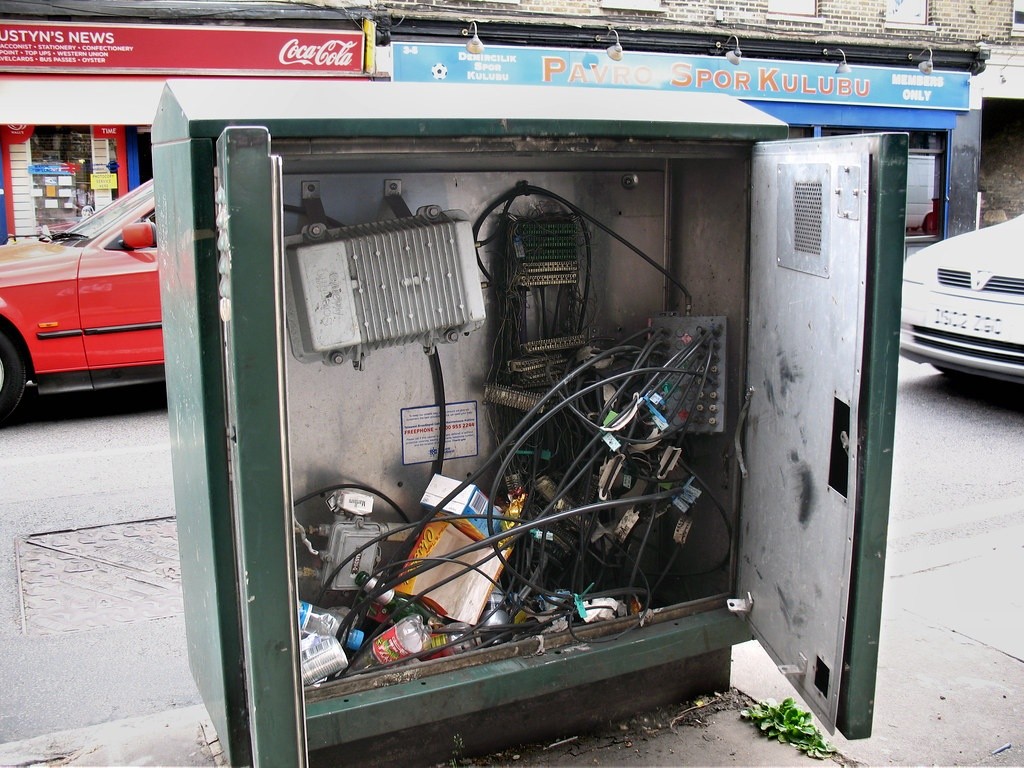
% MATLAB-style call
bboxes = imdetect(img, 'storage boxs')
[393,474,515,626]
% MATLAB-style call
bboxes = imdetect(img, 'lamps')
[715,35,742,65]
[908,48,934,75]
[462,22,484,55]
[595,30,623,61]
[823,48,853,74]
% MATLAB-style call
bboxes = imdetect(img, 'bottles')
[481,578,509,645]
[420,624,477,662]
[355,571,445,630]
[299,600,365,651]
[335,614,432,679]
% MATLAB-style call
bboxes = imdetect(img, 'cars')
[1,165,221,424]
[899,213,1023,390]
